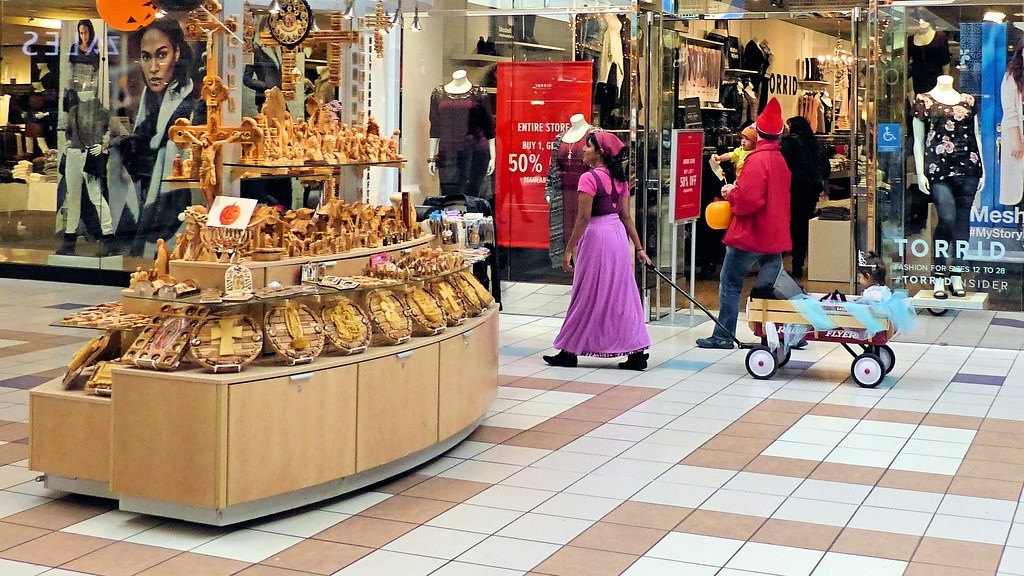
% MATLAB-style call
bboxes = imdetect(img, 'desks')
[0,181,58,212]
[809,217,856,283]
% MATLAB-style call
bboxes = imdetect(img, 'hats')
[741,122,757,144]
[756,96,784,135]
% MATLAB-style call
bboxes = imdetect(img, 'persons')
[243,15,290,197]
[302,66,336,107]
[379,295,399,329]
[181,205,207,261]
[854,251,894,304]
[185,131,240,212]
[428,69,497,197]
[906,22,951,140]
[7,55,58,160]
[678,43,720,89]
[914,74,985,299]
[68,20,99,95]
[332,302,360,342]
[999,38,1023,250]
[695,97,831,350]
[54,91,120,258]
[199,26,219,61]
[281,111,399,164]
[133,17,207,175]
[544,114,603,268]
[595,0,644,128]
[542,132,652,369]
[707,29,775,130]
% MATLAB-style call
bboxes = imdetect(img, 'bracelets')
[638,248,643,251]
[427,158,436,163]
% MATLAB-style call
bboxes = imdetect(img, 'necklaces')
[568,123,586,160]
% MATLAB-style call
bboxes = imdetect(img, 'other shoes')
[543,350,577,367]
[792,266,803,279]
[696,337,734,349]
[618,351,649,370]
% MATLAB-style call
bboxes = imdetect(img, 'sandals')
[947,275,966,297]
[933,273,948,299]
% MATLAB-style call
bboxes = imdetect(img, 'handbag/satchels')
[83,147,109,178]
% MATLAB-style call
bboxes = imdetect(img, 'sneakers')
[790,339,808,348]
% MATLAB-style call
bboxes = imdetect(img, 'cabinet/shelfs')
[451,40,566,94]
[28,159,501,527]
[676,34,865,139]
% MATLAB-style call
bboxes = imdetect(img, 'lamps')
[341,0,356,19]
[268,0,280,13]
[409,5,422,31]
[388,0,404,29]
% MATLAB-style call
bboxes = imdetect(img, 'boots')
[95,234,119,256]
[55,233,77,255]
